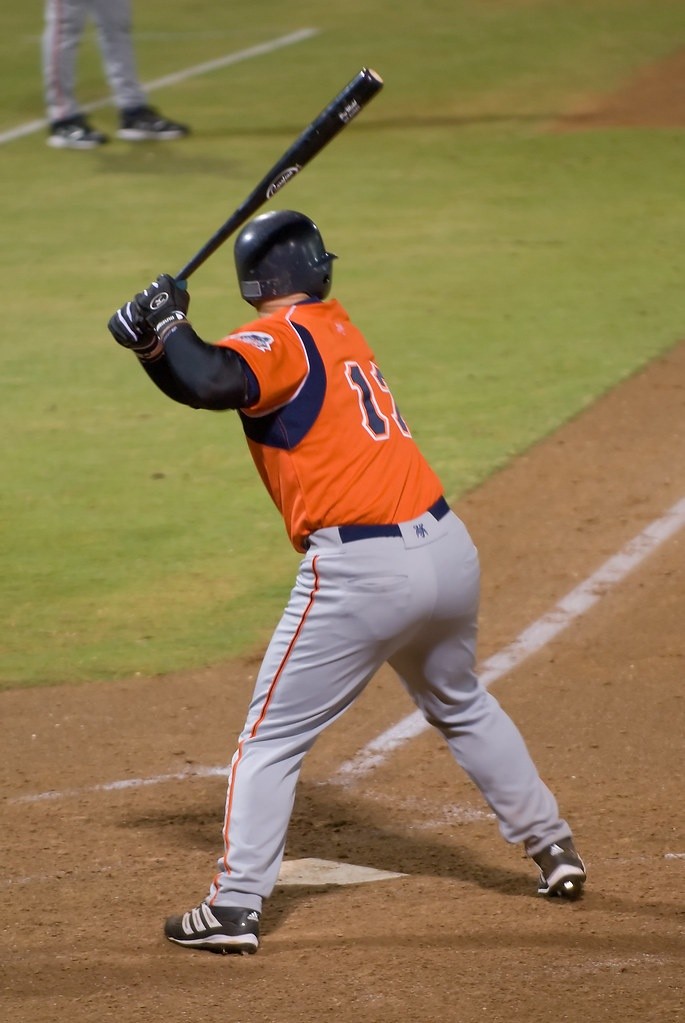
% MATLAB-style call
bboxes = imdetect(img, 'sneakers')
[47,114,111,150]
[118,105,188,140]
[165,901,260,956]
[532,838,587,897]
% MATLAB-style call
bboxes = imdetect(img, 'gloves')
[134,272,190,343]
[107,302,163,363]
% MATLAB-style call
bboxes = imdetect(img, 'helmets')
[233,209,338,305]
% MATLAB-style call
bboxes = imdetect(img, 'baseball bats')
[172,68,385,287]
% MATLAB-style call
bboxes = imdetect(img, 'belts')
[339,496,449,544]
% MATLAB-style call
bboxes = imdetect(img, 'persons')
[41,0,190,149]
[107,210,588,955]
